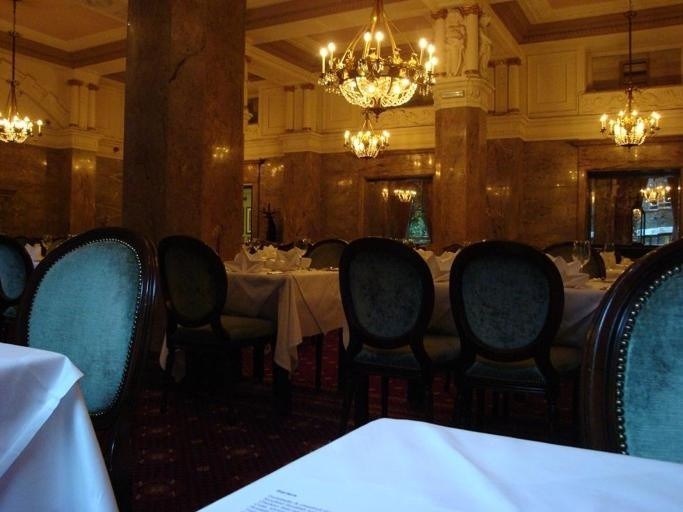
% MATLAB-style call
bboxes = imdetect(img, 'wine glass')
[570,238,591,274]
[602,241,614,270]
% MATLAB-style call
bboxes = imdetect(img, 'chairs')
[337,239,464,427]
[13,224,159,511]
[447,240,573,445]
[573,239,682,465]
[156,235,279,425]
[1,237,35,312]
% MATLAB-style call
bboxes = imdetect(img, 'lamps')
[317,0,441,109]
[340,109,391,161]
[0,0,45,144]
[639,176,672,207]
[599,0,663,148]
[393,188,417,204]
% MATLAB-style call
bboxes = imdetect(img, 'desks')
[216,253,637,389]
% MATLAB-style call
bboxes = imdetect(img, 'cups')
[251,238,261,251]
[43,234,52,254]
[301,257,312,270]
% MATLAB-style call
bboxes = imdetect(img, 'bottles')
[32,243,42,262]
